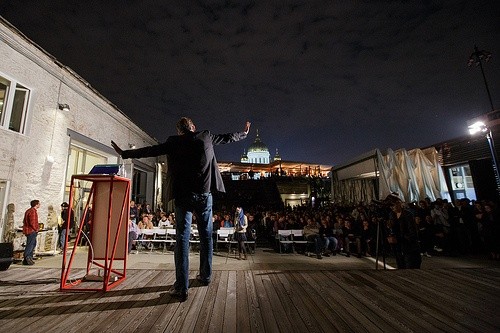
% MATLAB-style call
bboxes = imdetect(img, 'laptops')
[81,164,123,175]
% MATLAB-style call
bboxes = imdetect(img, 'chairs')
[132,227,255,254]
[278,229,310,255]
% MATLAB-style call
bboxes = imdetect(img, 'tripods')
[375,214,390,271]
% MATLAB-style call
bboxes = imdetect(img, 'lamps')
[129,144,135,149]
[59,104,70,112]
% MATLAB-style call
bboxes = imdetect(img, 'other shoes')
[169,286,188,301]
[239,255,241,260]
[317,255,322,259]
[196,274,210,284]
[347,251,351,255]
[424,252,432,258]
[324,253,330,257]
[243,256,248,260]
[434,245,443,252]
[365,251,369,256]
[333,251,335,256]
[23,259,35,266]
[59,250,63,254]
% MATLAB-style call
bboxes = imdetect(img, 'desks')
[13,228,59,255]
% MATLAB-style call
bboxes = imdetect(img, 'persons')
[57,202,75,255]
[22,200,41,266]
[128,191,500,269]
[111,117,251,301]
